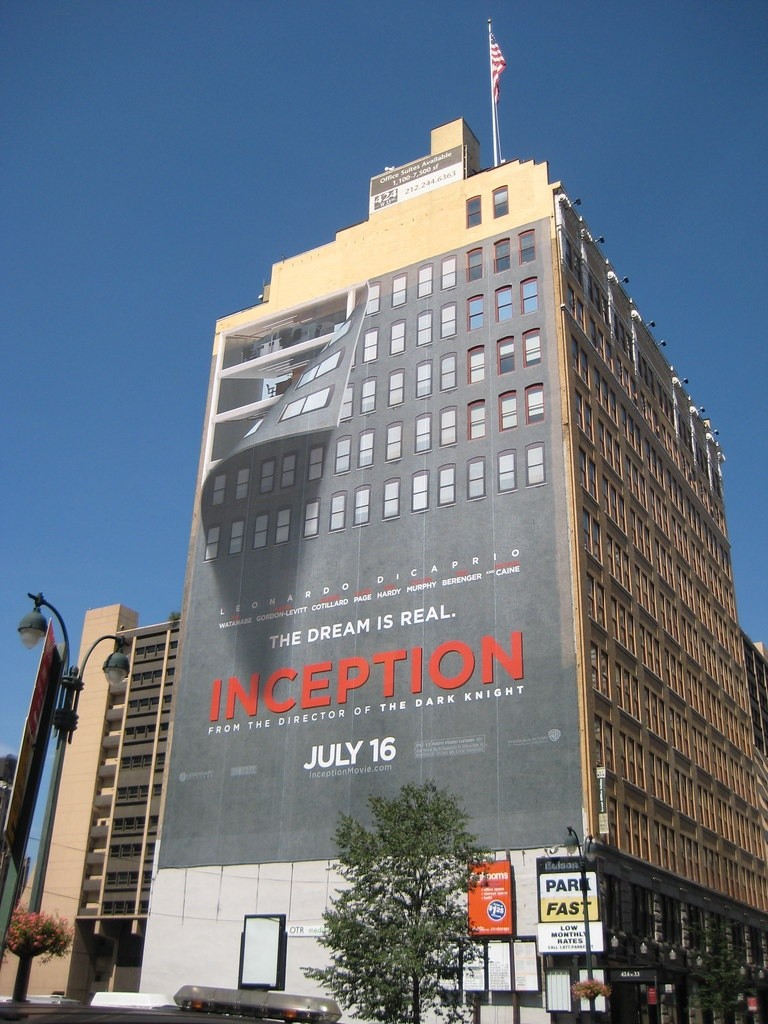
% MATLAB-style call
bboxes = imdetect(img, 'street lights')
[9,591,134,1002]
[564,822,605,1024]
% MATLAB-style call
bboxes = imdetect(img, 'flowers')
[2,899,76,966]
[569,977,612,1003]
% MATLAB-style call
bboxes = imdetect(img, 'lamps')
[681,378,689,384]
[658,340,666,347]
[697,406,705,412]
[621,277,629,283]
[646,321,655,327]
[595,236,605,243]
[572,197,581,206]
[712,429,719,435]
